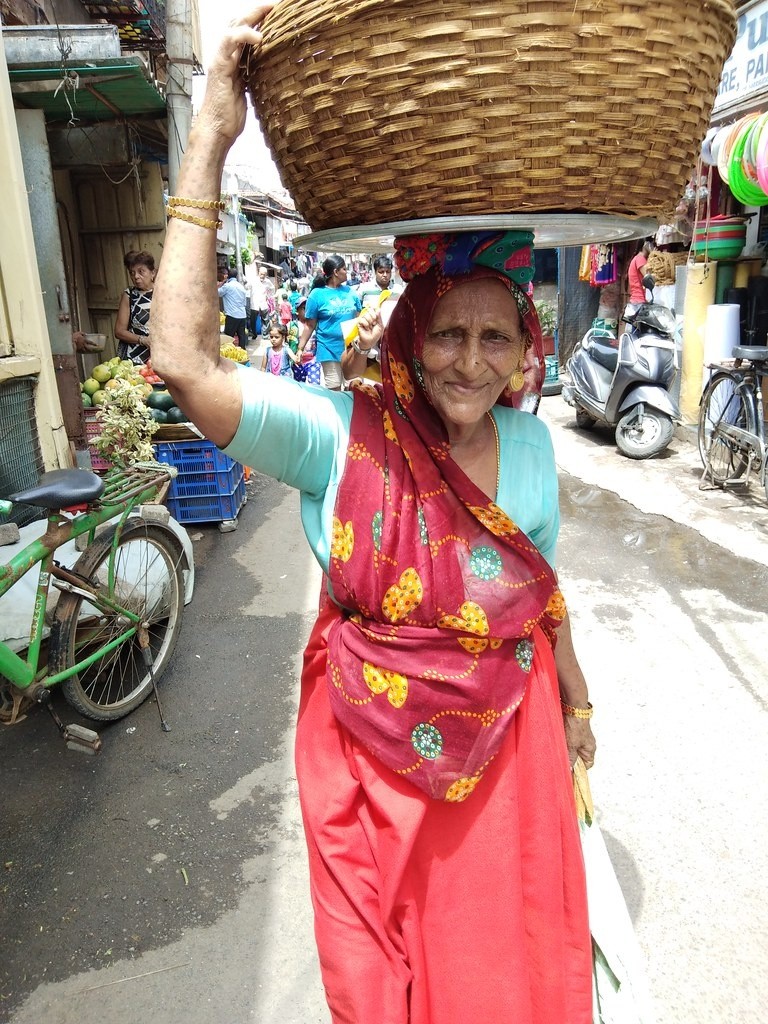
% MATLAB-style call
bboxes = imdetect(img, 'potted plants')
[534,300,557,356]
[89,360,171,506]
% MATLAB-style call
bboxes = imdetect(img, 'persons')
[72,330,98,353]
[150,3,609,1023]
[219,248,408,390]
[115,251,160,365]
[624,237,657,334]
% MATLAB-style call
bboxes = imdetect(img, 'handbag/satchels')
[116,338,128,359]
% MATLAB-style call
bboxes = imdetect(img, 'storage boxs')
[149,440,246,524]
[84,413,129,471]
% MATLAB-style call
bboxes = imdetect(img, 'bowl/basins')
[77,333,109,354]
[691,220,747,259]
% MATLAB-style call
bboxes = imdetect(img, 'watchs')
[353,335,371,356]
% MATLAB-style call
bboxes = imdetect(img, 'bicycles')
[0,461,198,758]
[696,345,768,506]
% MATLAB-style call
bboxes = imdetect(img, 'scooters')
[561,273,684,460]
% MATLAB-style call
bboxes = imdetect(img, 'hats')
[295,297,309,310]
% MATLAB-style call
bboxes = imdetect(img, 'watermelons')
[145,394,187,424]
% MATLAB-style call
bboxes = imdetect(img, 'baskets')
[238,0,738,222]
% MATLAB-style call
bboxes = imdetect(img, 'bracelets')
[163,193,225,211]
[164,205,223,230]
[138,335,144,346]
[560,698,593,719]
